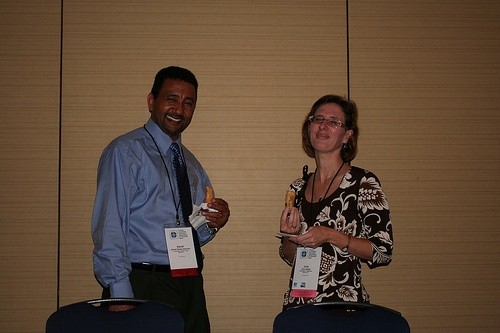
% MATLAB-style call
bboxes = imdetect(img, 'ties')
[169,142,203,274]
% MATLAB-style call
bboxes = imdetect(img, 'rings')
[292,225,296,228]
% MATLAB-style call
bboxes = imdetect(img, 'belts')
[131,262,171,272]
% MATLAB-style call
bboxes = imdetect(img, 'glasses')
[309,114,350,130]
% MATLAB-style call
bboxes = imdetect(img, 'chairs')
[45,298,184,333]
[272,301,410,333]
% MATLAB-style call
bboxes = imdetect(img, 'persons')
[91,66,230,333]
[279,94,393,310]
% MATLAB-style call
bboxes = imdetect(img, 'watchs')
[342,236,350,251]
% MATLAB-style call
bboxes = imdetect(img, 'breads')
[205,186,214,203]
[285,190,295,212]
